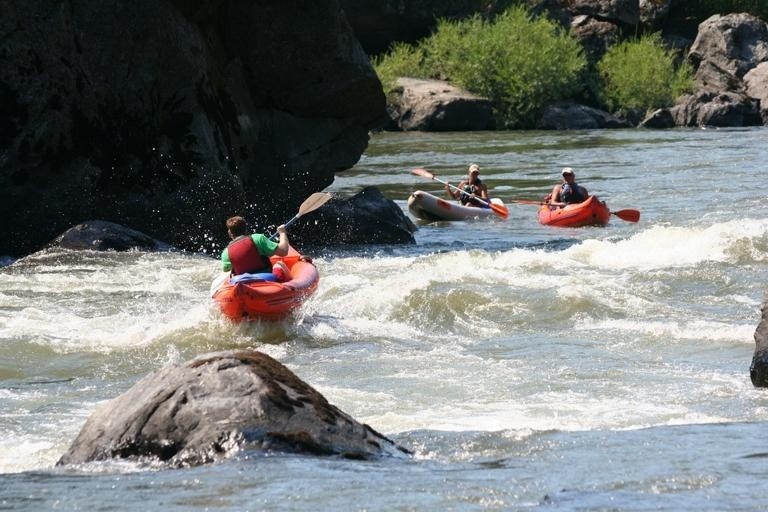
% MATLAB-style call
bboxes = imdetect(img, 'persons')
[549,167,588,208]
[444,163,492,209]
[220,215,291,285]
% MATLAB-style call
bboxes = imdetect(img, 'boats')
[532,194,613,228]
[208,233,322,322]
[406,187,511,224]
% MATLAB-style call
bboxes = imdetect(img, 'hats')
[562,167,574,175]
[469,164,480,173]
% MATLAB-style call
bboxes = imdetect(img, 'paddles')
[414,169,508,220]
[513,200,640,222]
[210,192,332,296]
